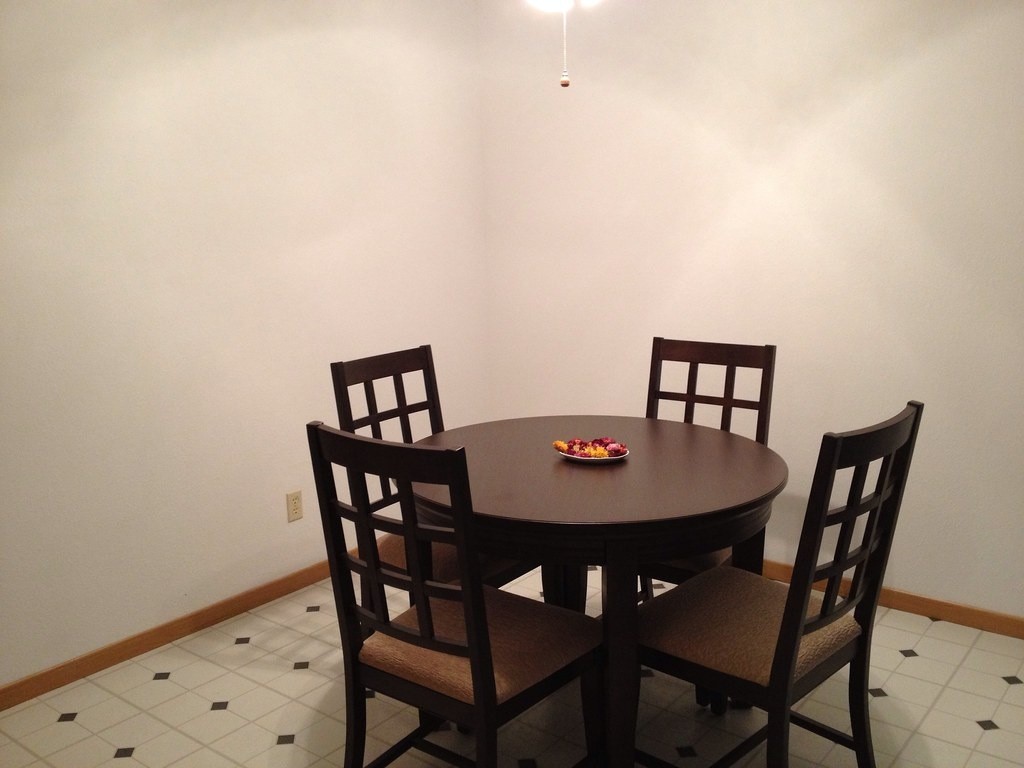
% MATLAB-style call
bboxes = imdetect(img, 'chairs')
[634,399,924,768]
[307,421,603,768]
[637,337,777,709]
[329,345,542,734]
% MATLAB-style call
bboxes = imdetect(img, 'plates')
[559,447,629,463]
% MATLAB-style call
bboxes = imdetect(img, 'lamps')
[527,0,603,89]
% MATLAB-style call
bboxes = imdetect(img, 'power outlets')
[287,490,303,523]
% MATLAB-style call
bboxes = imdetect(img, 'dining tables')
[390,415,788,768]
